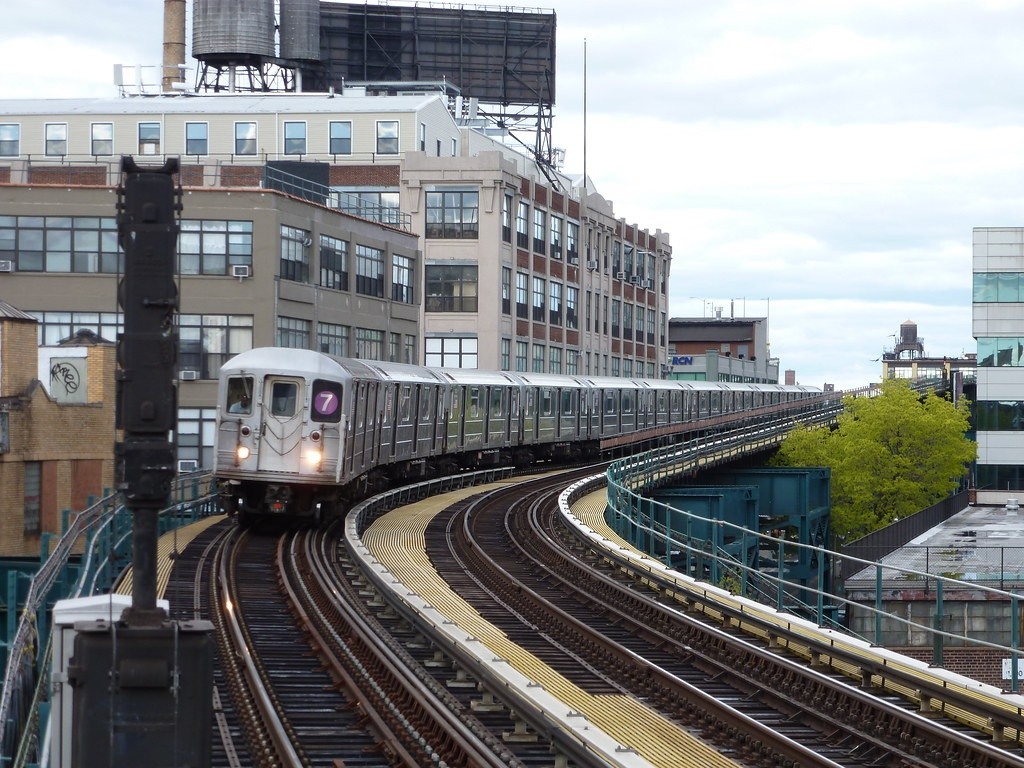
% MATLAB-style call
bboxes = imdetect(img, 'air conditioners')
[554,252,560,258]
[177,460,197,473]
[0,260,11,272]
[617,272,627,280]
[641,280,651,288]
[571,258,578,264]
[588,261,597,269]
[181,371,197,380]
[233,265,248,277]
[629,276,640,284]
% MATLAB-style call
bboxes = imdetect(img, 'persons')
[228,395,251,414]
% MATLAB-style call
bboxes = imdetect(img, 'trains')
[206,345,829,528]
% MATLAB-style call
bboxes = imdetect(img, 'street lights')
[761,297,769,343]
[689,296,705,317]
[707,302,714,318]
[736,296,745,317]
